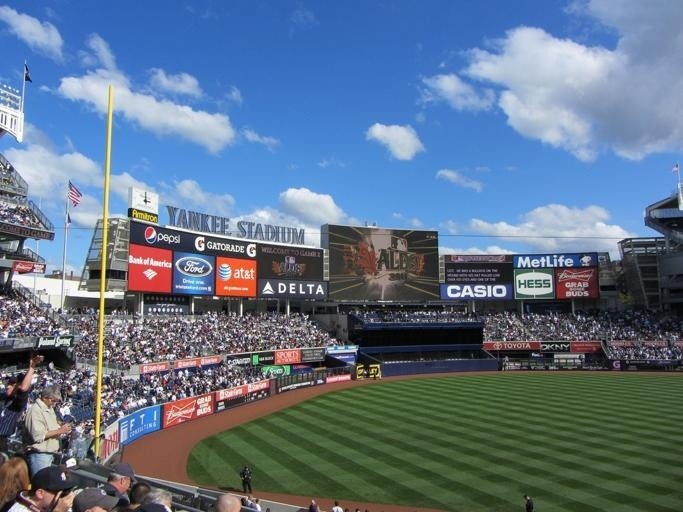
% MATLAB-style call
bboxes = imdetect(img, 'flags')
[67,182,84,208]
[672,164,679,172]
[24,62,33,82]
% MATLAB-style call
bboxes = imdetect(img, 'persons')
[0,159,18,189]
[1,285,682,511]
[0,201,44,228]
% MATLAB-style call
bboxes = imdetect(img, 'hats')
[101,484,129,507]
[32,466,78,492]
[72,488,120,512]
[111,462,138,482]
[138,503,172,512]
